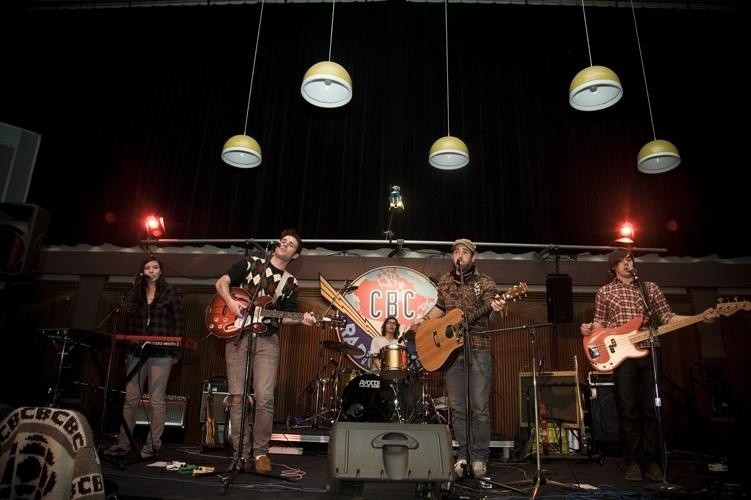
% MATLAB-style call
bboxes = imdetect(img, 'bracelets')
[425,315,430,319]
[422,317,427,321]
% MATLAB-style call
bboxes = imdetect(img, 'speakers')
[545,273,576,325]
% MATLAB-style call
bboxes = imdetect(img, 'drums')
[380,344,408,380]
[342,372,414,423]
[330,368,359,400]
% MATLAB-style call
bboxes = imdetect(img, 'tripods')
[94,281,138,471]
[292,350,350,431]
[193,253,290,493]
[447,263,528,500]
[391,366,447,425]
[506,329,590,498]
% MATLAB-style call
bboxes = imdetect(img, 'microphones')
[266,239,282,248]
[455,255,465,269]
[628,266,642,284]
[139,271,155,281]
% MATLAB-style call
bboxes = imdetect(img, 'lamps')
[383,185,405,242]
[631,0,682,173]
[614,221,636,250]
[427,1,470,169]
[223,2,269,169]
[144,215,166,240]
[302,1,353,110]
[568,1,624,110]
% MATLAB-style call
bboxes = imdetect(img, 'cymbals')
[320,340,364,355]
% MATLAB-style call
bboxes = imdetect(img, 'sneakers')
[228,458,248,471]
[644,463,665,482]
[471,461,487,476]
[254,456,272,474]
[453,459,470,477]
[141,445,159,458]
[625,464,643,481]
[103,444,129,456]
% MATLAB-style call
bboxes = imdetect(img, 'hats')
[451,239,476,255]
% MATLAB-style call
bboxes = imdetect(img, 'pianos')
[111,334,198,352]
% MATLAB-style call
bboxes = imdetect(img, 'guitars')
[539,358,548,455]
[415,282,528,372]
[205,286,348,338]
[583,296,751,373]
[203,384,218,451]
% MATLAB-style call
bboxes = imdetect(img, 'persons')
[101,256,187,459]
[370,317,408,371]
[413,238,507,477]
[214,229,317,474]
[580,248,719,482]
